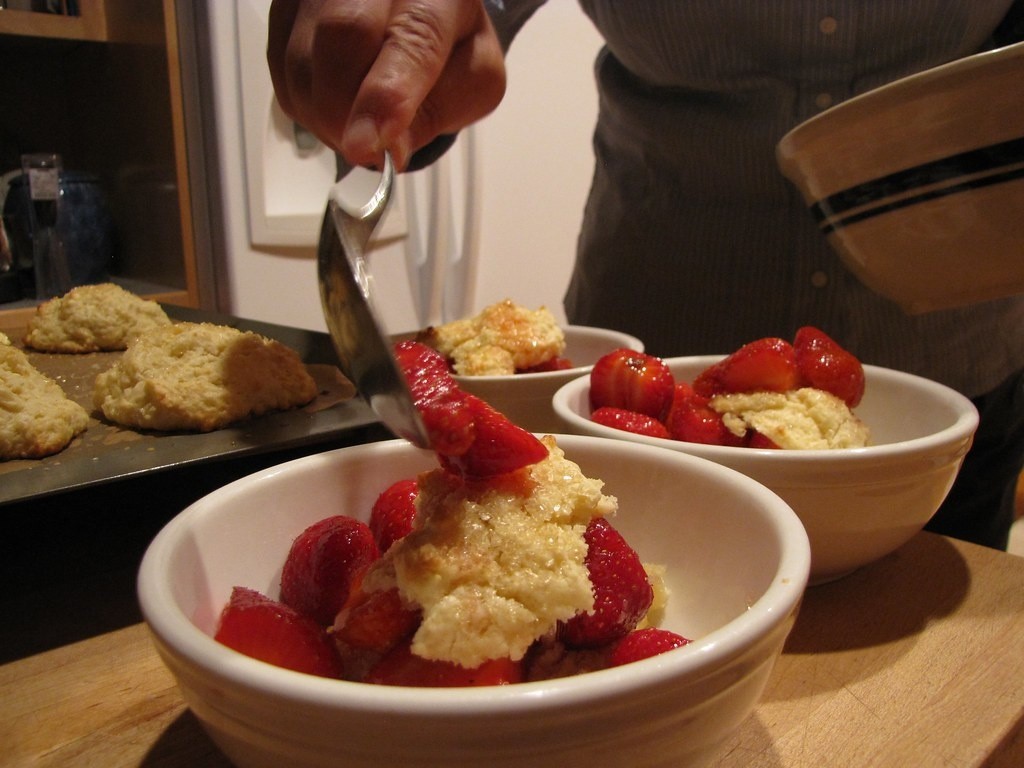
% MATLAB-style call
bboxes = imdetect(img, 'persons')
[265,1,1024,552]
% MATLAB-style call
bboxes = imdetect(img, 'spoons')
[311,144,426,455]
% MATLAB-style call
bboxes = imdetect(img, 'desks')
[0,525,1024,768]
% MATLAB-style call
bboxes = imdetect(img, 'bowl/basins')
[776,46,1024,318]
[137,322,980,768]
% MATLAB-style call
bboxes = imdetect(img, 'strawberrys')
[213,337,696,687]
[587,325,866,447]
